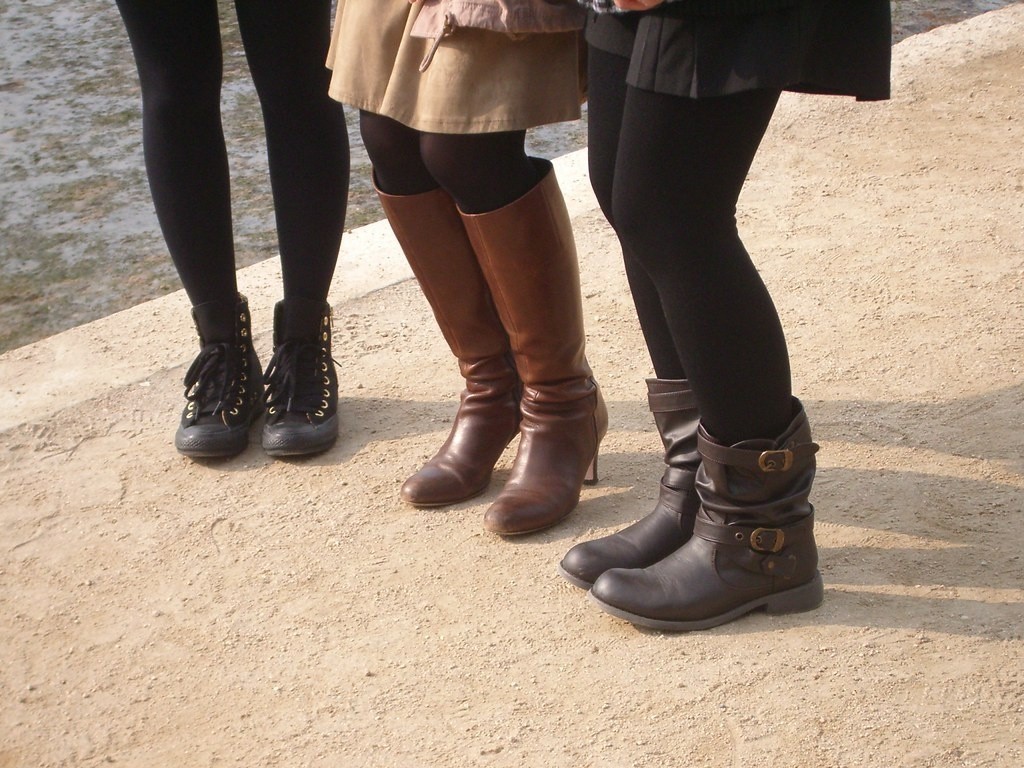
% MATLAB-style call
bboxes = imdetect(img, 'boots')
[586,396,824,631]
[370,162,522,506]
[556,378,700,592]
[454,157,608,536]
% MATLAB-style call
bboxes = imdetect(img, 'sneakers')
[174,292,266,458]
[262,299,343,456]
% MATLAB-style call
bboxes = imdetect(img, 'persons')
[114,0,350,458]
[325,0,609,534]
[560,0,892,631]
